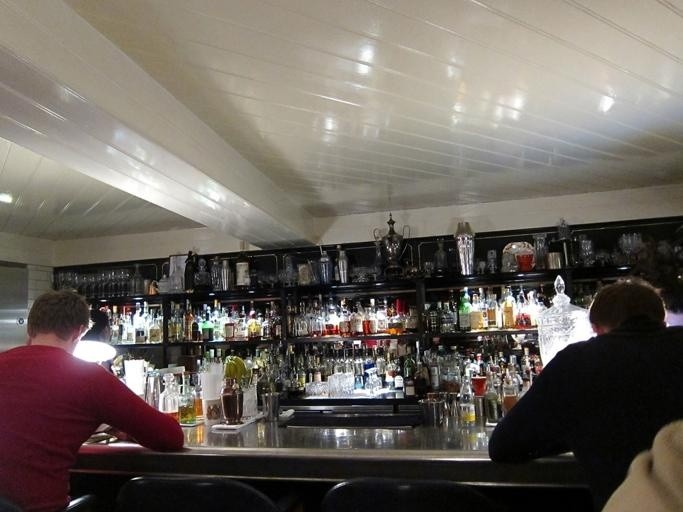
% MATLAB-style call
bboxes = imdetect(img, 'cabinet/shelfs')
[45,253,169,379]
[410,217,683,422]
[153,232,421,423]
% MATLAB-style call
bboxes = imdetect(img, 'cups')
[124,359,145,397]
[580,240,593,268]
[512,248,534,272]
[302,372,354,398]
[544,252,562,270]
[200,398,221,424]
[261,392,280,422]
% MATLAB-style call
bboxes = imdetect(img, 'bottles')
[286,293,406,337]
[158,372,195,425]
[315,244,348,285]
[129,263,145,298]
[192,256,230,292]
[439,282,528,333]
[194,339,398,384]
[403,340,540,399]
[432,243,447,273]
[89,298,280,345]
[456,377,475,429]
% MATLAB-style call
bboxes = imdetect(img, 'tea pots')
[152,275,169,294]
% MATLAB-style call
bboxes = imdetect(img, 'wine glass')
[618,232,642,265]
[57,268,128,300]
[364,368,381,396]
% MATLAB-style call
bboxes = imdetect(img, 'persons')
[83,309,116,372]
[488,281,682,508]
[0,290,183,511]
